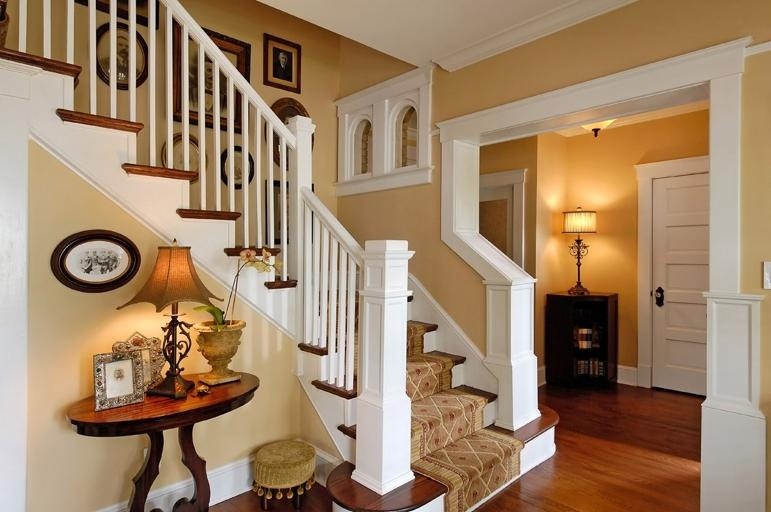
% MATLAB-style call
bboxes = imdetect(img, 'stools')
[252,441,317,511]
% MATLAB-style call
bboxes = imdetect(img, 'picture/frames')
[74,0,161,29]
[263,97,315,170]
[50,229,141,293]
[90,22,152,92]
[220,146,255,189]
[91,350,145,410]
[161,132,209,177]
[112,332,166,395]
[167,19,251,135]
[261,32,302,93]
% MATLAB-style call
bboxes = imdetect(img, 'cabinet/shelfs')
[544,293,619,394]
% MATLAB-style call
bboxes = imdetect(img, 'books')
[570,328,605,377]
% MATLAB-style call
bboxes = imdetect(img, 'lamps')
[117,236,225,399]
[562,207,597,297]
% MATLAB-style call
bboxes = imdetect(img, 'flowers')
[198,249,286,334]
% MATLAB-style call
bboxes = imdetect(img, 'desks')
[64,368,261,511]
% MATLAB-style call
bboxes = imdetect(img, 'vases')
[195,318,247,387]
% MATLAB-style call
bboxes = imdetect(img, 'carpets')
[345,318,526,512]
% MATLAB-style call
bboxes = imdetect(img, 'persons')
[99,31,140,80]
[190,57,227,114]
[273,52,291,79]
